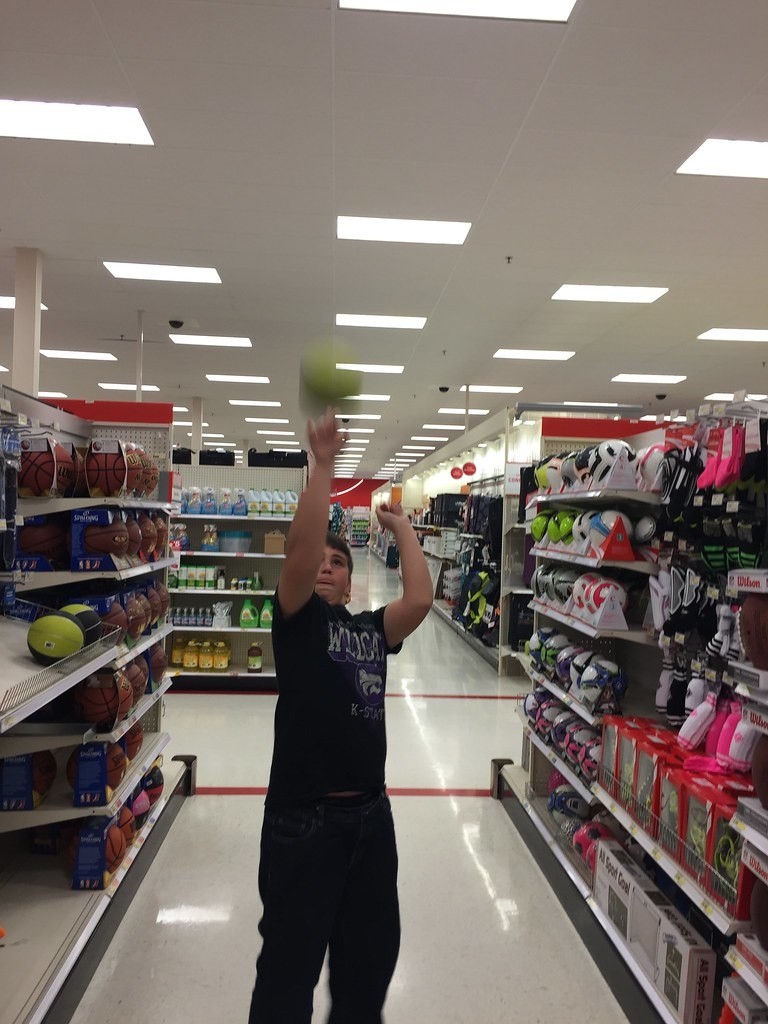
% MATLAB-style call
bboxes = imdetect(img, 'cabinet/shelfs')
[491,405,683,1024]
[587,400,768,1024]
[168,402,686,678]
[0,384,197,1024]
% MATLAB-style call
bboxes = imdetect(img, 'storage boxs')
[264,533,284,554]
[218,530,252,552]
[593,715,758,1024]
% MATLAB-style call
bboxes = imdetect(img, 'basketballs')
[16,435,171,892]
[298,334,364,404]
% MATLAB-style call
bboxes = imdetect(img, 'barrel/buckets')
[246,488,299,518]
[238,599,276,629]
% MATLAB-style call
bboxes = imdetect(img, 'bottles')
[170,636,264,675]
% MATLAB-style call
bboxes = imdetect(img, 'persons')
[244,401,435,1024]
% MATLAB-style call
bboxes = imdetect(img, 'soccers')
[520,437,672,874]
